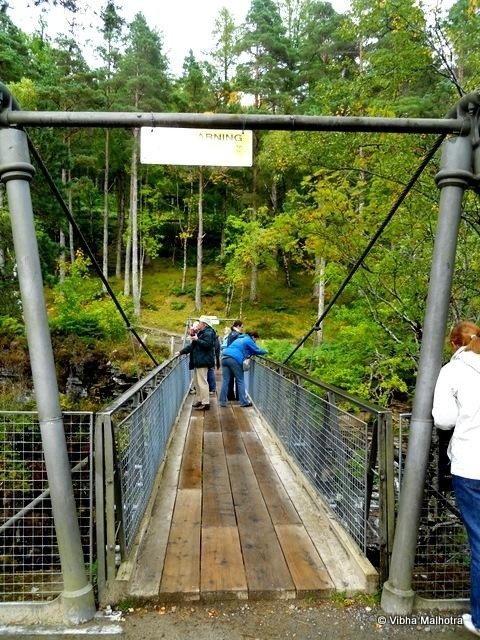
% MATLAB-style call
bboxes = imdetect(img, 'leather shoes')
[191,402,210,410]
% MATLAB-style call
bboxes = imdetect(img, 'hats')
[198,316,212,327]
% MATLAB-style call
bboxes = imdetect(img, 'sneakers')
[209,391,214,395]
[462,613,480,637]
[241,402,252,407]
[190,386,196,394]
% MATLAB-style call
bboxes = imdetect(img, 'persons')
[431,322,480,638]
[175,315,269,410]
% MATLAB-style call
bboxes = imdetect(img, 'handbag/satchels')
[243,359,251,371]
